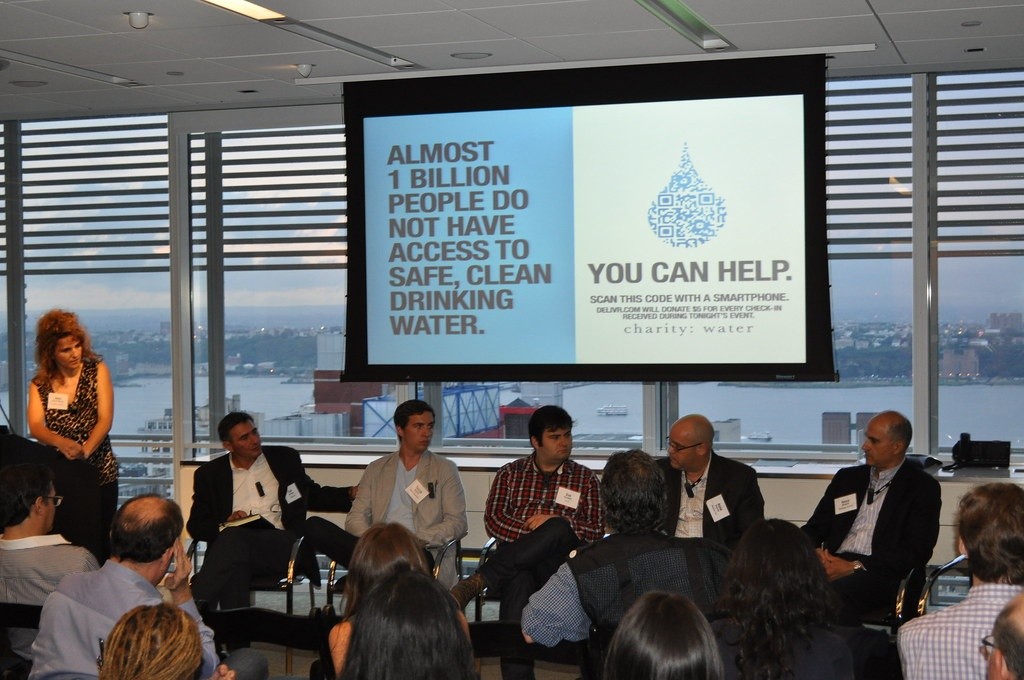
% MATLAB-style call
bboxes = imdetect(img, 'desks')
[178,448,1023,548]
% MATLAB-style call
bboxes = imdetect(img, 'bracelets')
[853,560,863,573]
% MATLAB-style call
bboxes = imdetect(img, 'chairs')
[0,496,1024,680]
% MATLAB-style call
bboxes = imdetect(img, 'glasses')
[36,495,63,507]
[982,634,1001,661]
[665,436,704,451]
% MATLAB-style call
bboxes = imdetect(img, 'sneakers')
[448,578,479,610]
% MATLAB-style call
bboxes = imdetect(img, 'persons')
[27,306,120,538]
[96,602,239,680]
[710,517,856,680]
[342,397,468,593]
[27,492,270,680]
[978,587,1024,680]
[652,415,765,552]
[340,571,477,680]
[519,443,737,655]
[601,589,727,680]
[896,480,1024,680]
[307,521,471,680]
[187,408,359,652]
[0,433,101,662]
[446,404,606,680]
[796,410,942,625]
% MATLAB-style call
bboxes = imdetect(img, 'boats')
[141,395,265,436]
[748,429,773,443]
[595,401,627,416]
